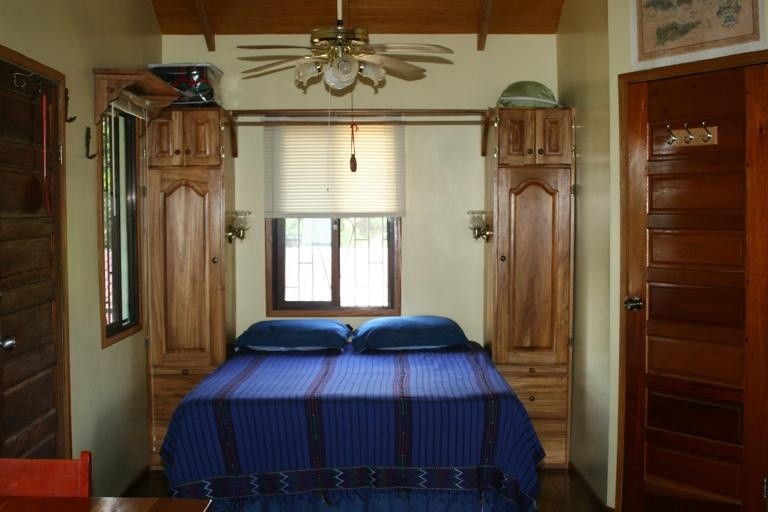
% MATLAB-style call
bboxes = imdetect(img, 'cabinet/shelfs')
[476,101,581,477]
[131,96,240,478]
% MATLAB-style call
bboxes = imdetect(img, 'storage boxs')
[146,62,224,104]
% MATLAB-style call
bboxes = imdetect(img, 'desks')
[0,493,215,512]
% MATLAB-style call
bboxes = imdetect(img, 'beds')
[153,346,551,512]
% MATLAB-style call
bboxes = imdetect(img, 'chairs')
[0,449,96,498]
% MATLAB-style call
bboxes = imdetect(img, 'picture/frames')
[635,0,761,61]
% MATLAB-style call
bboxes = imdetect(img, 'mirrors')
[91,66,182,350]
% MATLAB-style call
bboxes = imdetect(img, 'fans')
[232,0,459,83]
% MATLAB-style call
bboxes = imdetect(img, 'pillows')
[228,312,356,359]
[348,313,482,354]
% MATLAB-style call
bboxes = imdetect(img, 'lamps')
[222,203,252,244]
[291,49,391,177]
[462,207,493,244]
